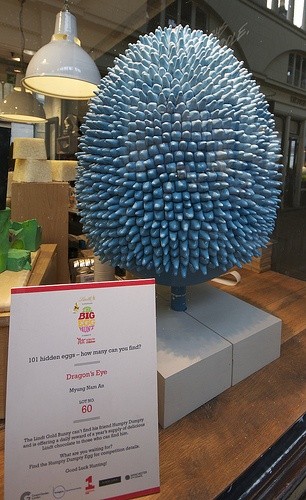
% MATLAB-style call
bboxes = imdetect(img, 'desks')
[128,263,305,499]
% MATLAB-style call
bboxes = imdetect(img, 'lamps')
[0,0,48,126]
[19,0,101,101]
